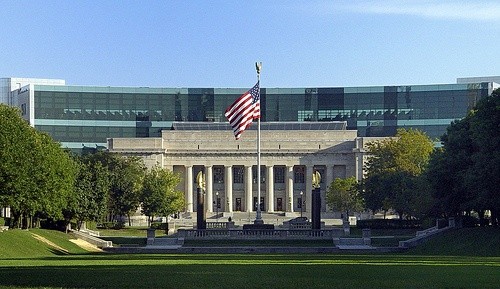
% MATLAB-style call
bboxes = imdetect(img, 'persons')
[157,215,163,222]
[401,213,408,220]
[106,211,122,223]
[226,217,233,235]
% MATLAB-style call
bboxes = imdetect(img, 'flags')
[224,80,260,141]
